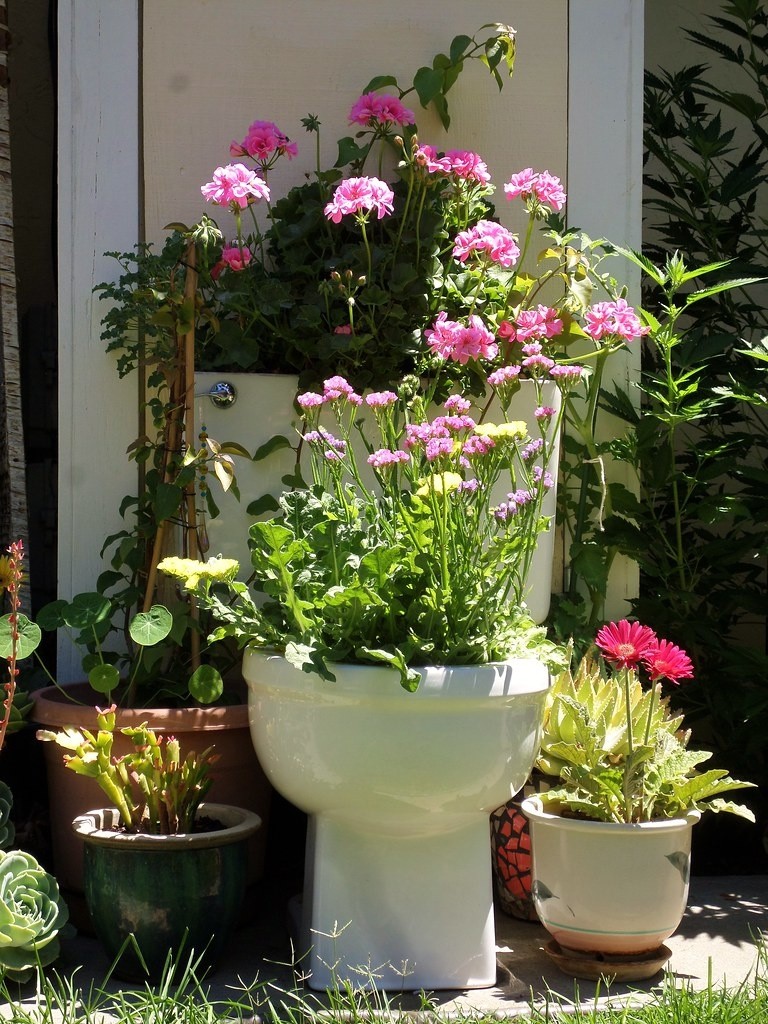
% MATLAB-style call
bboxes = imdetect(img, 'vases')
[492,764,702,982]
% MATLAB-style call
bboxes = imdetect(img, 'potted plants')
[0,564,273,982]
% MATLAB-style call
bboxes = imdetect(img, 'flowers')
[156,20,759,830]
